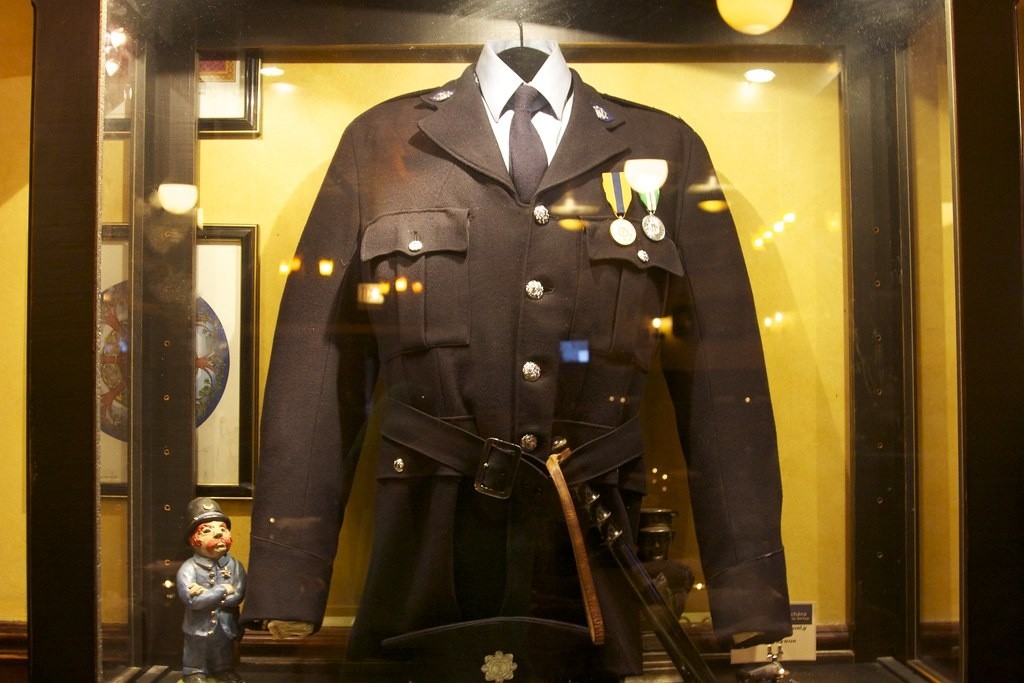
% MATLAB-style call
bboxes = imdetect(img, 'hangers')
[496,0,549,81]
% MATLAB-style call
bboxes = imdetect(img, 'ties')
[507,85,548,206]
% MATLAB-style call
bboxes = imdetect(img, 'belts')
[365,387,646,507]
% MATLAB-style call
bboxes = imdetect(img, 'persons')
[177,497,246,683]
[238,36,793,683]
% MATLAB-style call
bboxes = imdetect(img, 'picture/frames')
[103,17,136,137]
[98,224,131,499]
[194,225,262,499]
[196,52,263,136]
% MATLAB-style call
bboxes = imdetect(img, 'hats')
[380,616,611,683]
